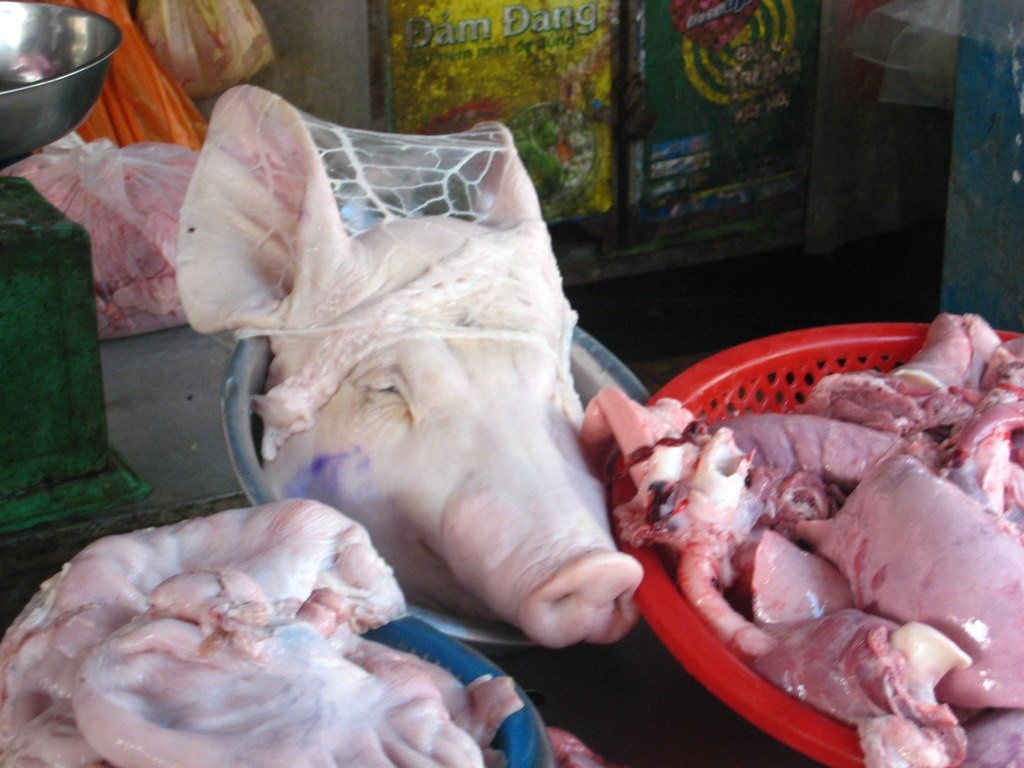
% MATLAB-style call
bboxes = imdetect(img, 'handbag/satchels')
[36,0,207,154]
[0,131,200,341]
[135,0,276,102]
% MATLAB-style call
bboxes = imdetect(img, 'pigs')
[171,86,643,647]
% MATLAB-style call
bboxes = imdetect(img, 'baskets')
[363,615,555,768]
[616,321,1024,768]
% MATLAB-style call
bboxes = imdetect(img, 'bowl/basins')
[220,325,657,654]
